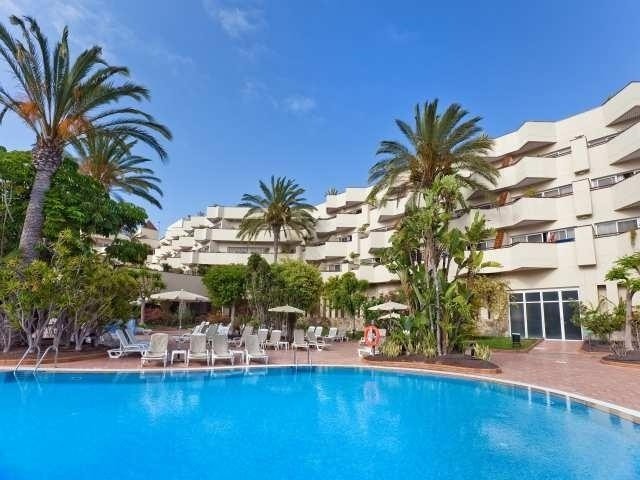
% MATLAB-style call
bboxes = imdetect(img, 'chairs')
[106,321,387,367]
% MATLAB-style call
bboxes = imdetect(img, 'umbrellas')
[266,302,305,336]
[129,296,151,323]
[377,311,402,331]
[367,299,410,331]
[149,289,211,328]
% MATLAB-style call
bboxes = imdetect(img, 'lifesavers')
[364,326,380,346]
